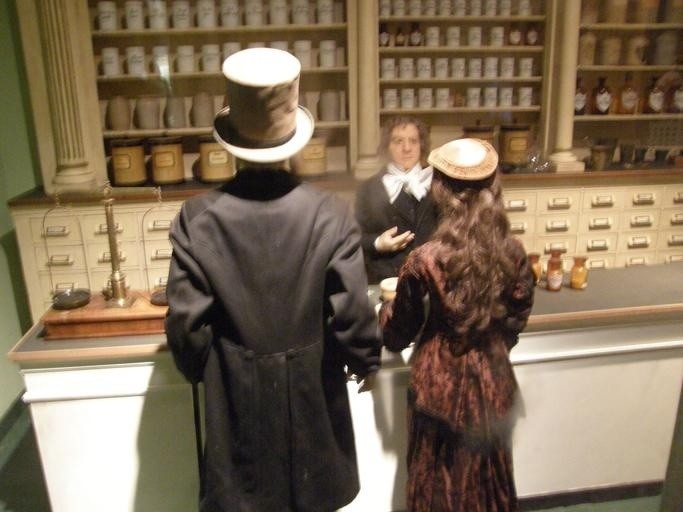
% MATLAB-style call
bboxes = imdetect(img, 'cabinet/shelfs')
[88,0,351,190]
[378,0,556,179]
[7,175,683,323]
[570,0,683,175]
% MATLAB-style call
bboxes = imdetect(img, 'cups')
[576,0,682,68]
[379,276,401,299]
[620,143,668,168]
[96,0,344,133]
[426,25,504,48]
[380,0,533,20]
[380,58,533,108]
[590,144,615,171]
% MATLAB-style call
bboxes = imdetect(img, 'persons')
[160,41,384,512]
[352,110,442,284]
[373,133,531,512]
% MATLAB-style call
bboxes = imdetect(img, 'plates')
[655,71,682,112]
[52,289,91,308]
[150,288,169,304]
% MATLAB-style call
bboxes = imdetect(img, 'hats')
[428,137,499,180]
[212,46,315,164]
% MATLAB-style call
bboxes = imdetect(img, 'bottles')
[643,74,664,114]
[409,23,422,47]
[617,72,639,114]
[394,25,405,47]
[525,23,538,45]
[379,23,390,47]
[672,85,682,113]
[593,75,613,114]
[527,249,588,292]
[509,22,521,46]
[572,78,588,114]
